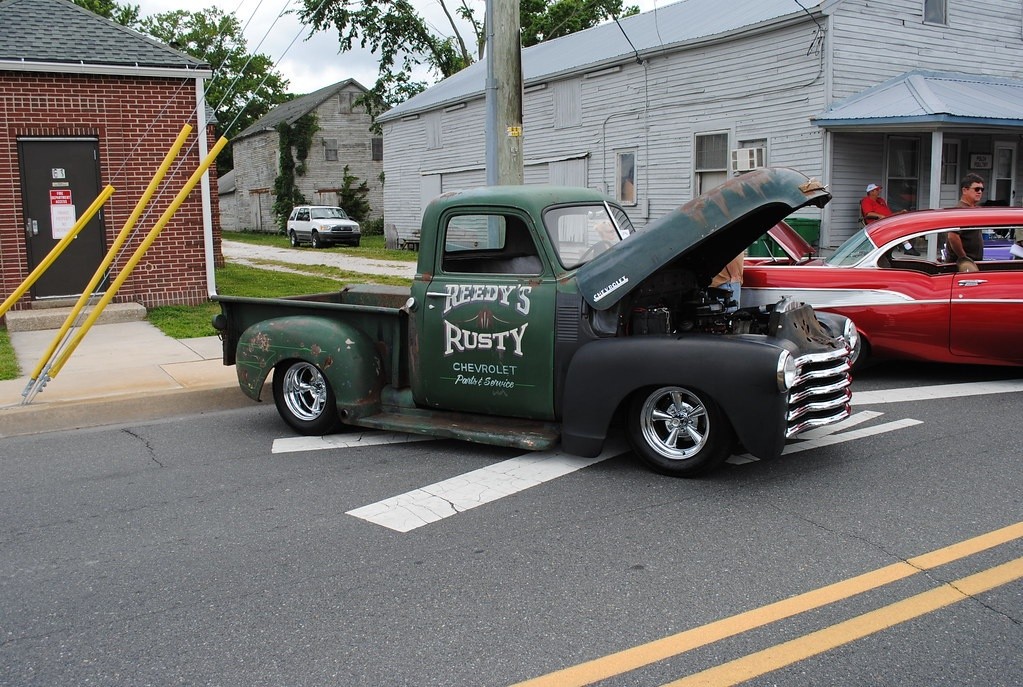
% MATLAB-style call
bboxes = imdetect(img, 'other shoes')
[904,246,921,256]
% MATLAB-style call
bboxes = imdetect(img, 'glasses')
[966,186,984,193]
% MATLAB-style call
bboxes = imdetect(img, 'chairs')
[857,200,878,229]
[482,253,544,276]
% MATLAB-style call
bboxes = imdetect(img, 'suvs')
[287,205,361,249]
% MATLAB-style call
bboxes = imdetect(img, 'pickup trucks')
[209,165,854,479]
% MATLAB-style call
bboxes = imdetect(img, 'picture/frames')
[969,152,993,170]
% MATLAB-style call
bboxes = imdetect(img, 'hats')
[866,184,883,192]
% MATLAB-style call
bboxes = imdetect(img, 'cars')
[739,207,1022,379]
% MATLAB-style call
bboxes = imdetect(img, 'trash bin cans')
[749,217,822,259]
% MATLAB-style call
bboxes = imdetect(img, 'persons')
[595,221,618,258]
[945,174,984,262]
[708,251,744,312]
[861,184,921,256]
